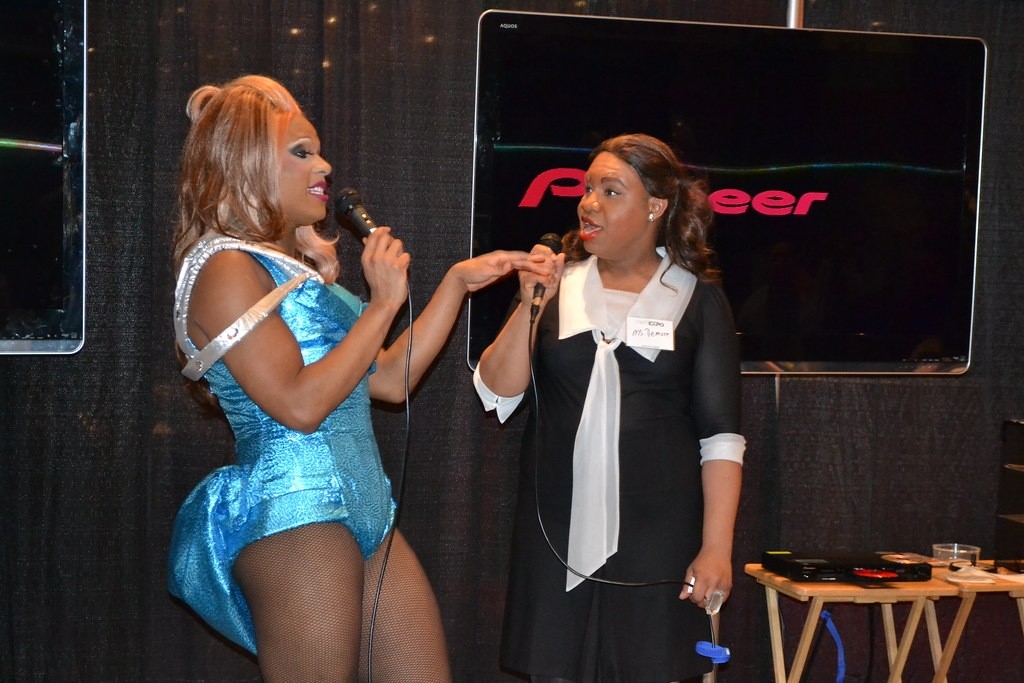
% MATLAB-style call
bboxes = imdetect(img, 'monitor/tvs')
[0,0,89,355]
[467,7,989,375]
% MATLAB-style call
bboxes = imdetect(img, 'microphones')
[533,233,564,324]
[333,187,377,241]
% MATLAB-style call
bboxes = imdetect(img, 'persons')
[166,75,550,683]
[471,135,746,683]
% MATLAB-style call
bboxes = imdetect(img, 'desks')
[743,557,1024,683]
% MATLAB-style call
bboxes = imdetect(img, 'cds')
[881,554,926,564]
